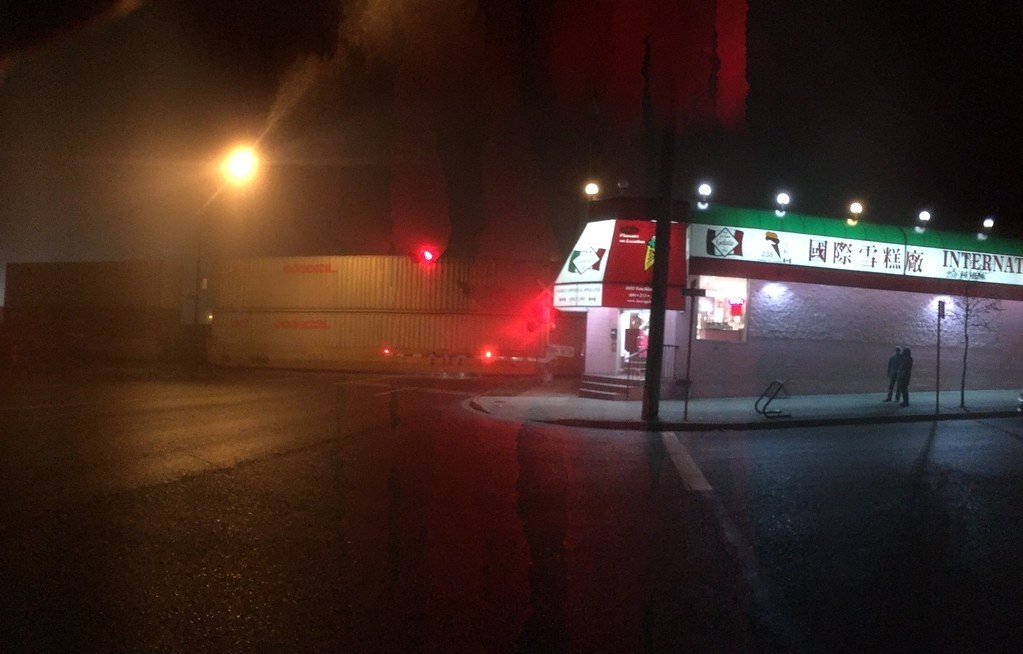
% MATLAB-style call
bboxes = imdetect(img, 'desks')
[699,329,741,341]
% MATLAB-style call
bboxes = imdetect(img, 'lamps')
[698,184,712,202]
[917,211,931,229]
[777,193,790,210]
[618,179,629,197]
[982,218,995,235]
[850,203,864,221]
[585,184,599,201]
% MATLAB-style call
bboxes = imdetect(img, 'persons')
[882,346,913,407]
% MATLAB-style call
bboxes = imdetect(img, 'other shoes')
[900,402,909,407]
[883,398,891,402]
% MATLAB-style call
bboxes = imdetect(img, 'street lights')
[192,145,258,375]
